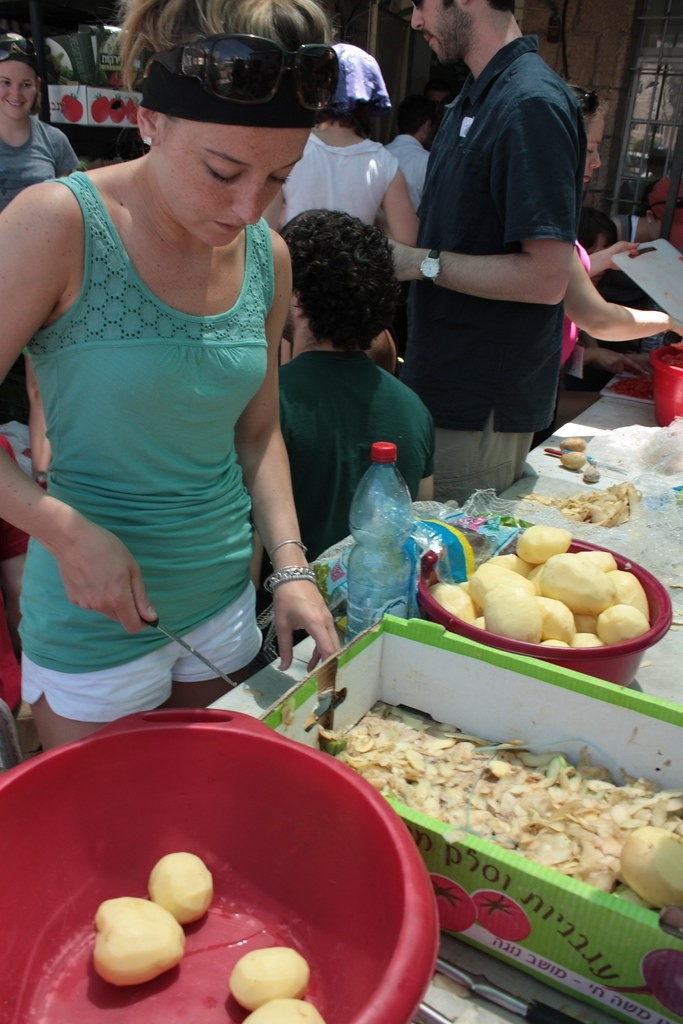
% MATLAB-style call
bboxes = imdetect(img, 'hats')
[649,179,683,248]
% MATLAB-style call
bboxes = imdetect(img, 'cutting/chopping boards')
[609,237,683,322]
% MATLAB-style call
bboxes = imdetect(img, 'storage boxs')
[48,85,143,128]
[259,614,683,1024]
[46,31,146,90]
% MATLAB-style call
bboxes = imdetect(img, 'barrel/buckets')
[647,345,683,426]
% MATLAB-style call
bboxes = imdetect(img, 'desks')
[205,396,683,1024]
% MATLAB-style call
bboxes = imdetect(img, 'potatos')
[427,526,653,647]
[560,452,586,469]
[92,852,325,1024]
[559,437,586,452]
[619,824,683,910]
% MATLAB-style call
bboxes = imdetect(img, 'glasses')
[577,90,600,112]
[0,37,37,61]
[650,197,683,209]
[142,33,340,110]
[411,0,422,9]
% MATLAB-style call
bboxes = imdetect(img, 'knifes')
[141,616,238,689]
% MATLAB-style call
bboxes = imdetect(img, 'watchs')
[419,249,442,283]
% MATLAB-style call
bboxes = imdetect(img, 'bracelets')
[263,565,318,593]
[270,538,308,560]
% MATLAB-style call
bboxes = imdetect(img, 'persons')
[549,82,682,438]
[379,1,587,502]
[381,78,460,237]
[256,42,423,244]
[249,208,436,618]
[0,0,344,748]
[0,33,80,712]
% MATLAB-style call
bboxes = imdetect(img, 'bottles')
[344,441,419,646]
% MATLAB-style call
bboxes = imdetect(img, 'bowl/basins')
[0,706,442,1024]
[411,538,674,687]
